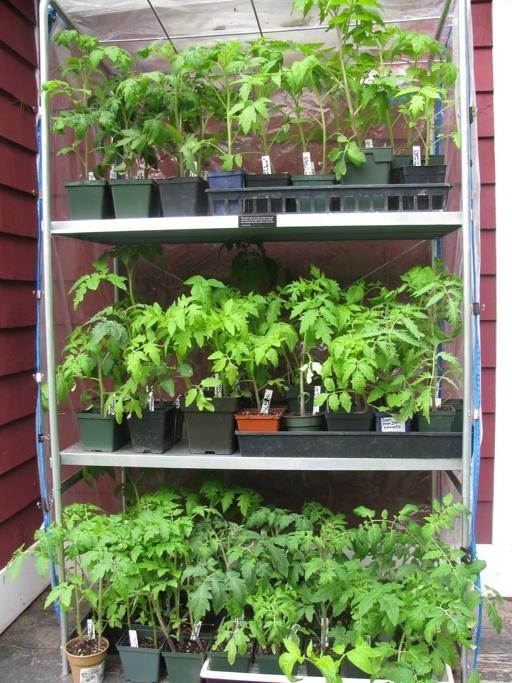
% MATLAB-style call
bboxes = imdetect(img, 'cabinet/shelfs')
[33,0,482,681]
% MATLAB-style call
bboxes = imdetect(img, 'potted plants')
[40,236,461,458]
[43,5,455,220]
[4,467,505,682]
